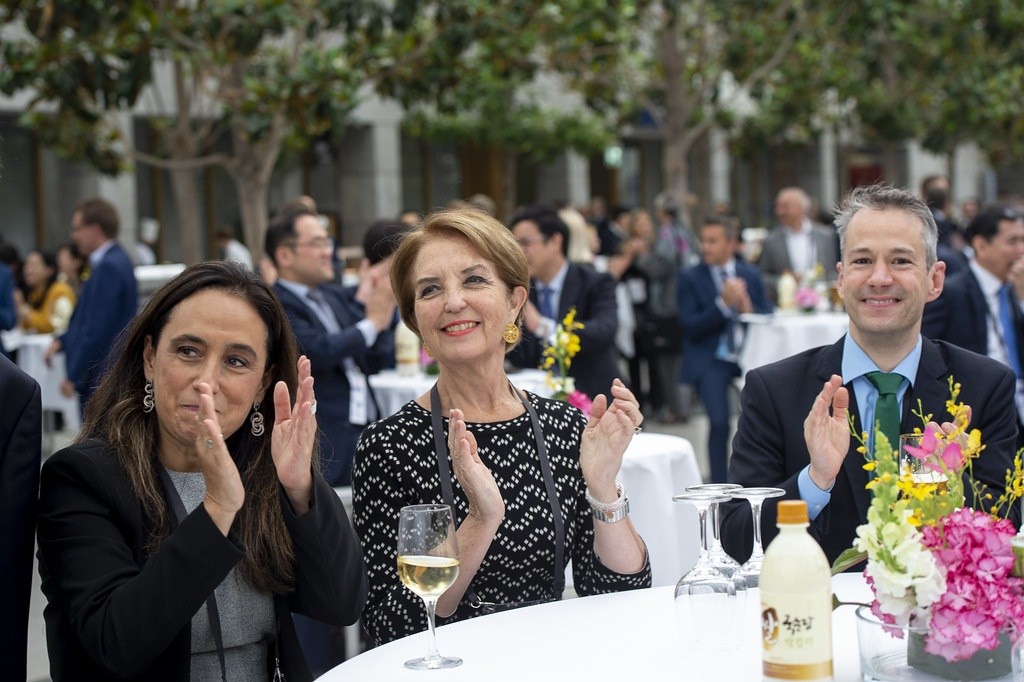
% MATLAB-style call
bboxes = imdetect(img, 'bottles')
[777,269,845,317]
[759,499,833,681]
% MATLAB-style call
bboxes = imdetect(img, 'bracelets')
[585,481,626,510]
[535,317,547,337]
[591,494,629,523]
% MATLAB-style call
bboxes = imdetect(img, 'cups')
[899,432,952,495]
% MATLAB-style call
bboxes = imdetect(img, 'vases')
[854,601,1024,682]
[906,605,1021,681]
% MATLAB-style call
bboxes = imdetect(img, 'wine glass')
[397,504,463,671]
[672,484,787,609]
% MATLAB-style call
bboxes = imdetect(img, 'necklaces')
[431,375,565,608]
[151,450,286,682]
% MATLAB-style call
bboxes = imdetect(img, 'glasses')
[517,235,544,247]
[279,236,333,249]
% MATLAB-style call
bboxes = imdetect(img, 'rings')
[204,434,224,448]
[307,400,317,414]
[633,426,643,435]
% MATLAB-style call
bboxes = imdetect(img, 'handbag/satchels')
[455,596,557,622]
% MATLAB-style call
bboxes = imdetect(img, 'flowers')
[540,306,592,414]
[794,264,825,308]
[831,374,1024,663]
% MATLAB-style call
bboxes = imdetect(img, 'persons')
[36,260,367,682]
[719,183,1024,573]
[0,173,1024,487]
[0,352,42,682]
[352,208,653,654]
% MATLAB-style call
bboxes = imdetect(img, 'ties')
[996,284,1020,377]
[539,286,560,376]
[863,372,906,501]
[719,271,735,354]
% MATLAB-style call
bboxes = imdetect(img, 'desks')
[13,331,78,456]
[561,432,713,597]
[735,313,850,390]
[367,368,574,421]
[315,571,1024,682]
